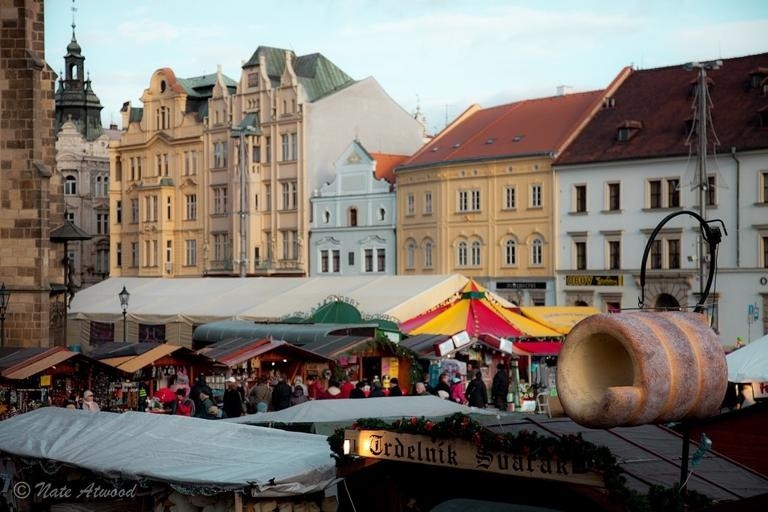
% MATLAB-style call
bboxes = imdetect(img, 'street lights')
[116,285,131,342]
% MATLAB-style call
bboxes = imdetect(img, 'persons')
[1,359,511,420]
